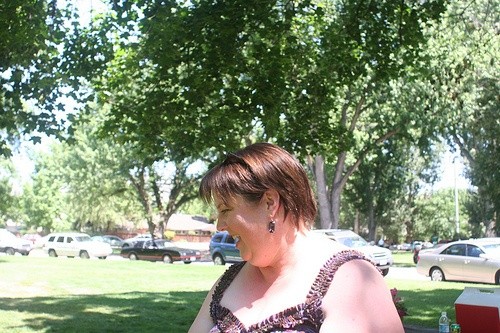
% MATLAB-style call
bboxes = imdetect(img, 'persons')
[378,238,384,247]
[414,243,422,263]
[188,143,405,333]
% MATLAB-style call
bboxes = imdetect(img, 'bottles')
[439,312,451,333]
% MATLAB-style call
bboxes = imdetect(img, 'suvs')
[313,228,392,277]
[209,231,246,266]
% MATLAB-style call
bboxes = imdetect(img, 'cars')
[42,233,112,261]
[0,229,32,256]
[22,233,49,247]
[121,238,202,264]
[122,233,159,247]
[416,238,500,285]
[95,234,131,247]
[399,240,433,251]
